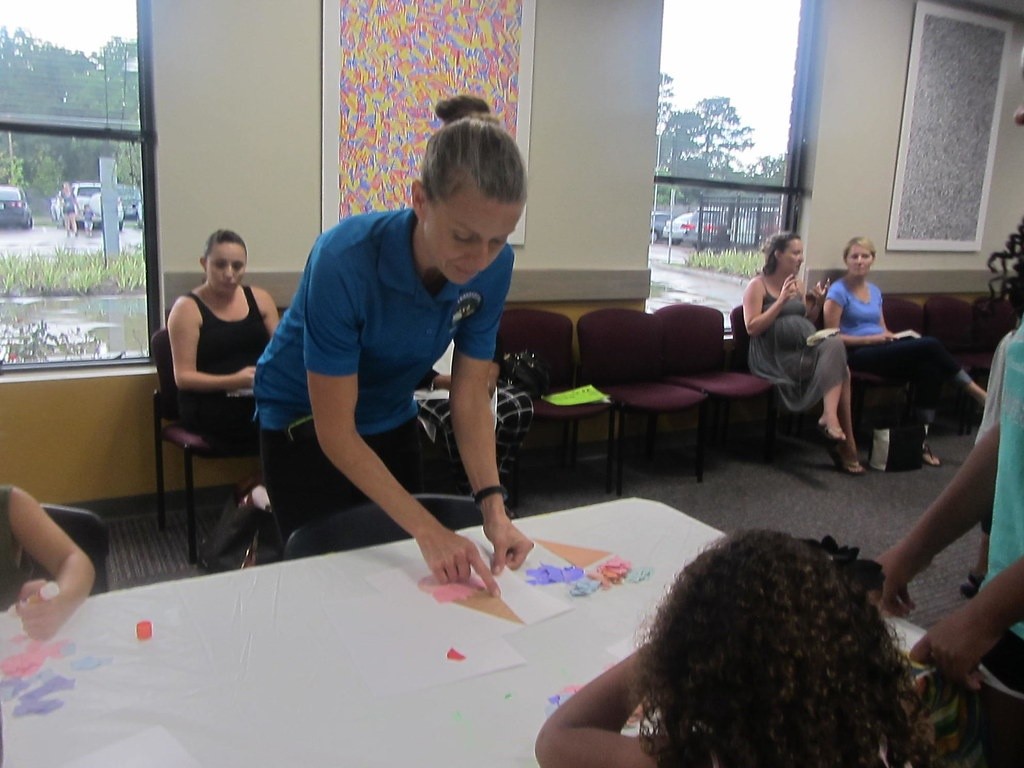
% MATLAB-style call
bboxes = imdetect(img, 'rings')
[891,338,893,342]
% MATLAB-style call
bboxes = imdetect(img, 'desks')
[0,496,928,768]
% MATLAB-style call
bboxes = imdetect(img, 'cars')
[0,184,32,230]
[730,206,781,249]
[650,212,680,246]
[132,192,144,228]
[685,207,738,250]
[115,183,141,220]
[662,213,699,248]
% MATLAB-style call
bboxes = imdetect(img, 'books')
[894,329,922,340]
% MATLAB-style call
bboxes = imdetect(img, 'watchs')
[470,483,508,509]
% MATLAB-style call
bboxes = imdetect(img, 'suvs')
[50,180,125,231]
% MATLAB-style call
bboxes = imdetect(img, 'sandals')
[975,407,983,417]
[960,573,983,598]
[922,444,942,466]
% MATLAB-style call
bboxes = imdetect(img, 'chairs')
[152,292,1021,562]
[15,502,107,607]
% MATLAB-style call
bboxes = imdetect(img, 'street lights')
[652,121,666,245]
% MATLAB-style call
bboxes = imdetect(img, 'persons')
[824,237,988,466]
[0,483,95,638]
[167,95,535,598]
[743,232,867,476]
[868,218,1024,768]
[535,524,946,768]
[62,181,95,238]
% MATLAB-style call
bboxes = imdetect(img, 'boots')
[200,501,258,574]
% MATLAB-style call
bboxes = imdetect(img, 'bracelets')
[430,373,439,393]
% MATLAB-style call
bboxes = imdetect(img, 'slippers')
[830,447,864,475]
[816,426,844,443]
